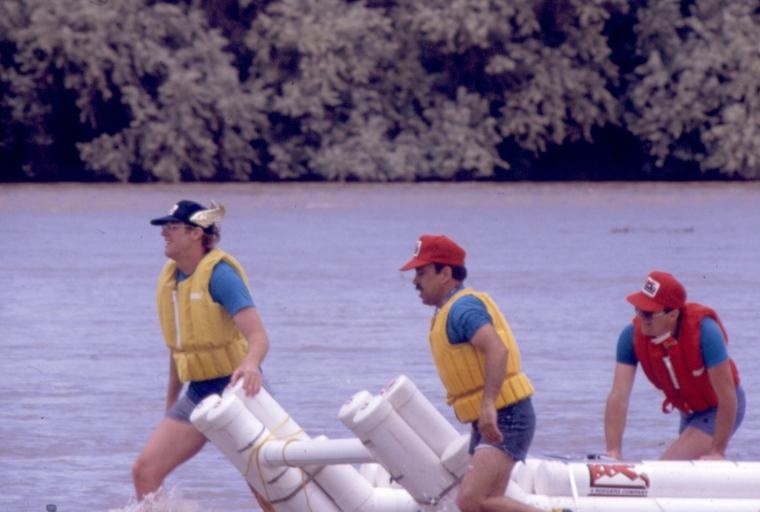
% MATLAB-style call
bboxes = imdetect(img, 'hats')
[626,273,688,312]
[150,199,224,234]
[399,235,466,273]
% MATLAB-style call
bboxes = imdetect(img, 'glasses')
[635,308,665,320]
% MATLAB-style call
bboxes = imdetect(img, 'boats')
[189,378,760,512]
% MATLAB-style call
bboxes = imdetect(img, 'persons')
[397,235,575,512]
[130,199,278,512]
[605,270,747,465]
